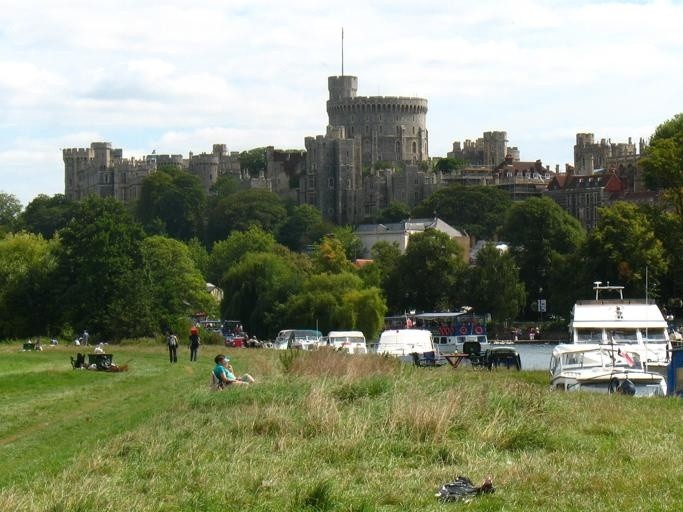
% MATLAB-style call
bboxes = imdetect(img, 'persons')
[168,332,178,362]
[187,330,199,361]
[668,322,683,347]
[196,322,261,349]
[51,336,57,344]
[83,330,88,345]
[512,327,538,341]
[223,358,233,374]
[74,338,80,346]
[215,355,254,388]
[400,317,445,329]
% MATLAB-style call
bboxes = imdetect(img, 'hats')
[221,355,230,363]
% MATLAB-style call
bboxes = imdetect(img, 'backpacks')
[168,335,176,347]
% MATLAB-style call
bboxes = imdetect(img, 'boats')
[568,263,673,387]
[539,341,668,396]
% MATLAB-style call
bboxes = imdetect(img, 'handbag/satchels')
[211,370,236,385]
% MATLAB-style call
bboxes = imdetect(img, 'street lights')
[537,287,543,339]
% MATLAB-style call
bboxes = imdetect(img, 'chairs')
[209,368,250,394]
[221,332,525,375]
[67,348,119,373]
